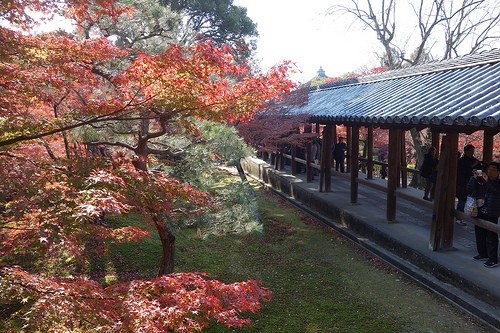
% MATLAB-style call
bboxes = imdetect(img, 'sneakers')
[472,254,489,261]
[484,261,499,268]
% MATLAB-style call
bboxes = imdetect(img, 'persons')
[455,144,481,225]
[422,145,439,202]
[382,160,387,179]
[268,136,322,180]
[407,155,416,178]
[467,162,500,268]
[334,137,347,173]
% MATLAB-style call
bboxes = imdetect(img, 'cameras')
[477,170,483,176]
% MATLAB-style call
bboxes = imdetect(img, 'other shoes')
[457,219,466,226]
[423,196,432,201]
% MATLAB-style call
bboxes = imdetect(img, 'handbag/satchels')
[465,196,478,217]
